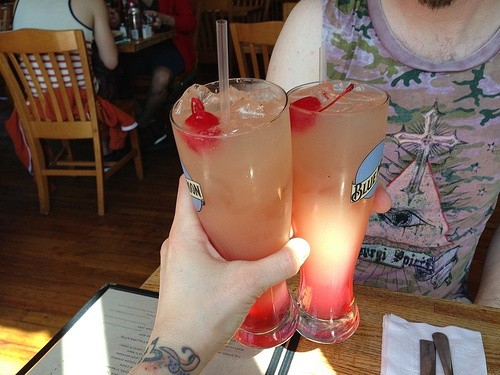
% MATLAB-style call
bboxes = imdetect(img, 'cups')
[169,76,299,349]
[287,80,390,347]
[141,17,153,39]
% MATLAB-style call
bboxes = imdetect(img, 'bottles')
[127,0,140,40]
[120,23,127,38]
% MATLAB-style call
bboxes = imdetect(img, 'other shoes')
[133,119,175,154]
[103,146,131,172]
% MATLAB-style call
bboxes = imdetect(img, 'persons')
[264,0,500,309]
[127,173,309,375]
[12,0,151,195]
[102,0,197,148]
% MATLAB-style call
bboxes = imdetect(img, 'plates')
[112,30,123,37]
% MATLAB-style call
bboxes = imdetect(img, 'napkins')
[379,313,487,375]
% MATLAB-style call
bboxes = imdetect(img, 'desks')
[139,265,500,375]
[117,29,175,53]
[202,5,263,16]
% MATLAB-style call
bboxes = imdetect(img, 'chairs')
[0,0,298,216]
